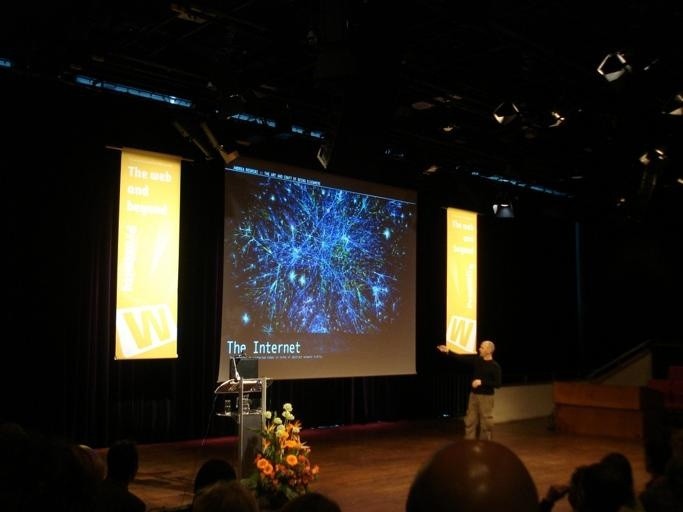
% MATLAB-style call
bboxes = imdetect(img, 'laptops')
[230,358,258,378]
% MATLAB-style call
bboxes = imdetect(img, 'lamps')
[174,120,216,161]
[198,112,240,164]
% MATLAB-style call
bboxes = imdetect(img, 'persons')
[1,440,146,511]
[436,340,501,440]
[168,461,341,512]
[406,439,539,511]
[538,452,644,512]
